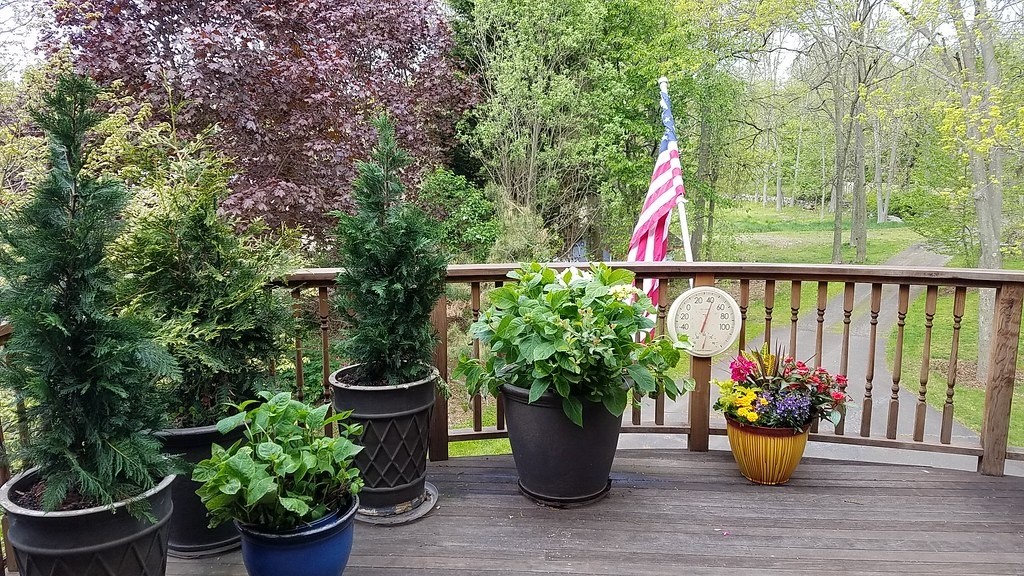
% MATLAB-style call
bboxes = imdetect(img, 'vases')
[723,410,811,486]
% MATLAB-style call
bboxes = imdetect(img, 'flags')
[615,82,688,370]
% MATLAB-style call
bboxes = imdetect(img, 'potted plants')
[452,257,696,510]
[0,62,194,576]
[98,118,300,561]
[191,389,365,576]
[325,108,499,527]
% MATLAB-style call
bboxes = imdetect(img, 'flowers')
[708,337,858,434]
